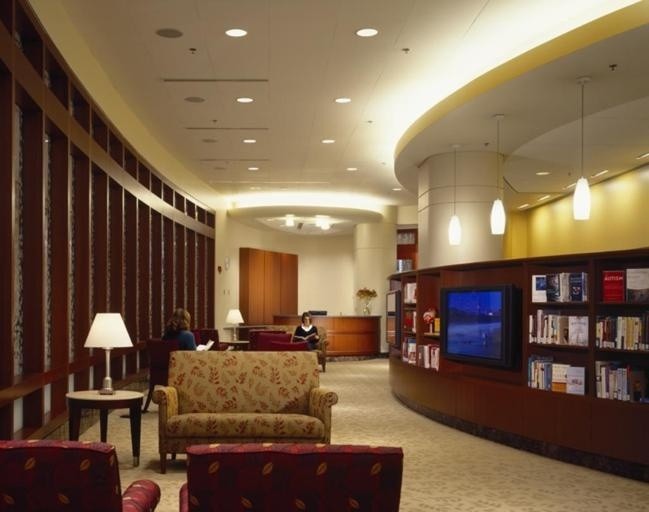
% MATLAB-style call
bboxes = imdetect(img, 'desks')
[64,389,145,468]
[219,339,251,351]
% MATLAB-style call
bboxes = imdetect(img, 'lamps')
[488,114,509,239]
[572,76,592,224]
[225,309,245,340]
[446,145,468,247]
[285,211,333,232]
[81,309,136,395]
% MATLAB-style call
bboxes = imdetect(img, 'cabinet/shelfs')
[399,272,445,372]
[594,256,649,407]
[239,246,300,326]
[526,260,591,400]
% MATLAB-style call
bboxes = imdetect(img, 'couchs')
[289,323,330,373]
[150,347,340,475]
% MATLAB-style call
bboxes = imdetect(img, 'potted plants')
[355,287,377,315]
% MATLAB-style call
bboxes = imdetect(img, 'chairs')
[270,339,308,350]
[308,310,329,316]
[256,332,292,350]
[197,326,229,351]
[178,442,403,512]
[139,336,184,415]
[1,439,164,511]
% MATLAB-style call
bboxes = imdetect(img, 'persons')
[162,308,198,351]
[293,312,319,351]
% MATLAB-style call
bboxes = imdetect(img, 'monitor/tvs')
[386,291,401,346]
[440,284,522,372]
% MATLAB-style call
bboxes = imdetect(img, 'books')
[528,267,649,403]
[402,282,440,372]
[293,333,316,343]
